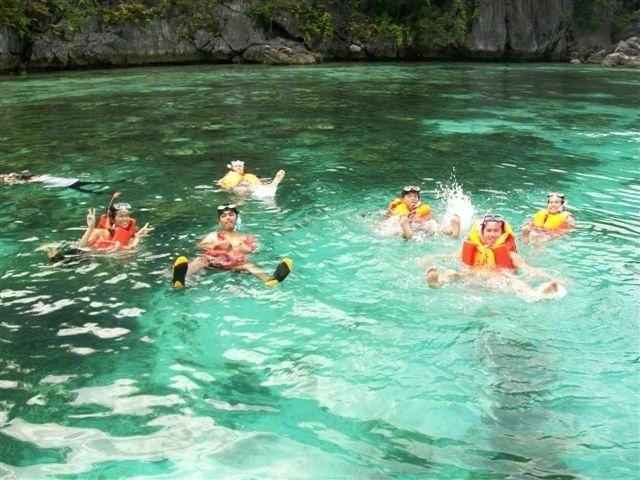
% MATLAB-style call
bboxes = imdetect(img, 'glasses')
[111,202,132,217]
[217,204,238,217]
[482,215,504,227]
[548,191,564,203]
[231,160,244,169]
[402,186,420,197]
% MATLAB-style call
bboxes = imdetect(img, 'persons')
[48,202,155,268]
[420,214,571,300]
[383,186,460,239]
[218,160,285,195]
[9,170,126,195]
[522,192,576,245]
[172,203,293,289]
